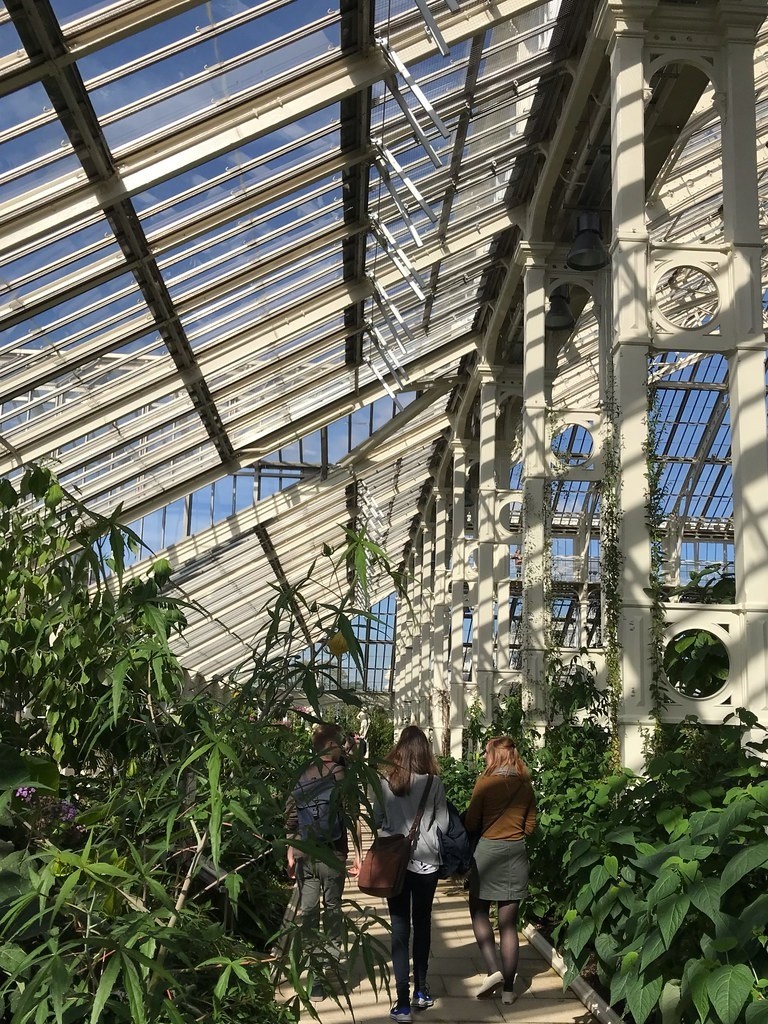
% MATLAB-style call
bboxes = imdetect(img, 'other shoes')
[310,985,326,1001]
[323,966,342,992]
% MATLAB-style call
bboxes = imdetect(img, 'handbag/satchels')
[357,834,411,898]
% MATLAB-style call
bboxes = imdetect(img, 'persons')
[465,736,537,1005]
[373,726,449,1023]
[354,707,371,738]
[343,733,367,759]
[284,722,364,1002]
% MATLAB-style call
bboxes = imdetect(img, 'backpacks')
[290,764,347,847]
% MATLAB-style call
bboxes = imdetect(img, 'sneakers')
[389,1005,412,1023]
[411,992,433,1008]
[502,991,518,1005]
[476,971,506,998]
